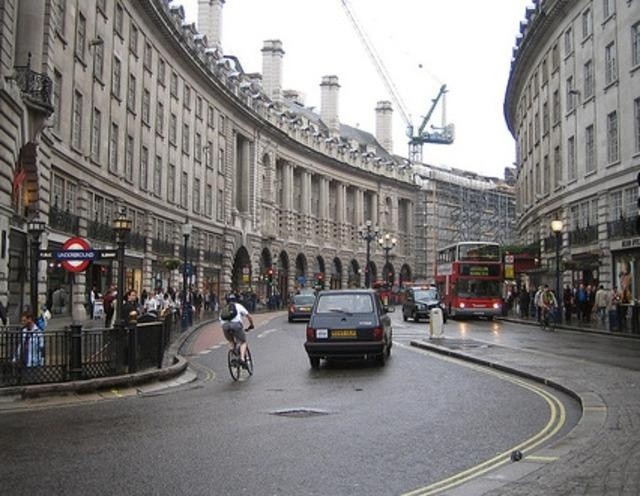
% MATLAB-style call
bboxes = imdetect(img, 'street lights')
[28,209,44,321]
[378,234,397,286]
[182,216,191,329]
[552,212,563,304]
[357,220,379,289]
[114,205,132,374]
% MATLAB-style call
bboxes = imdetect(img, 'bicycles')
[227,326,253,381]
[538,305,559,333]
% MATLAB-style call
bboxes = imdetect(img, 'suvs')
[403,286,446,323]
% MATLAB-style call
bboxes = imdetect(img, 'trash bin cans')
[608,309,621,331]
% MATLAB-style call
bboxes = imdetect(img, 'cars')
[305,289,395,367]
[288,287,318,322]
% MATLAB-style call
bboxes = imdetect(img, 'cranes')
[340,0,454,161]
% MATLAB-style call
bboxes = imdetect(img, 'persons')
[218,292,254,366]
[11,311,45,403]
[502,279,621,324]
[101,283,283,330]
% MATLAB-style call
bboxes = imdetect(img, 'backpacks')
[219,302,238,320]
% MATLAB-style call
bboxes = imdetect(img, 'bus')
[435,241,505,319]
